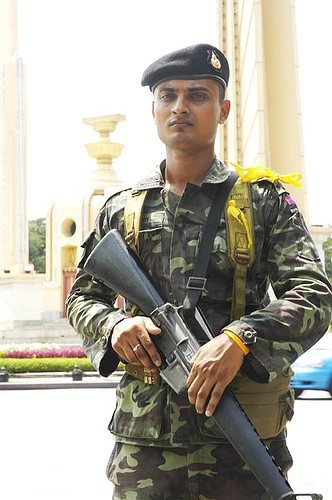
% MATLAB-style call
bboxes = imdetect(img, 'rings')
[132,343,141,352]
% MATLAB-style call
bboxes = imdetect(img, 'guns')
[81,227,324,500]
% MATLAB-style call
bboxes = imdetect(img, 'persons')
[63,43,332,500]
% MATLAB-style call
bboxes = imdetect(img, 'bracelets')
[224,330,250,356]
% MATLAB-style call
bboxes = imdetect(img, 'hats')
[141,44,230,94]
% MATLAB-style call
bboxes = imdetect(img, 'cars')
[289,331,332,398]
